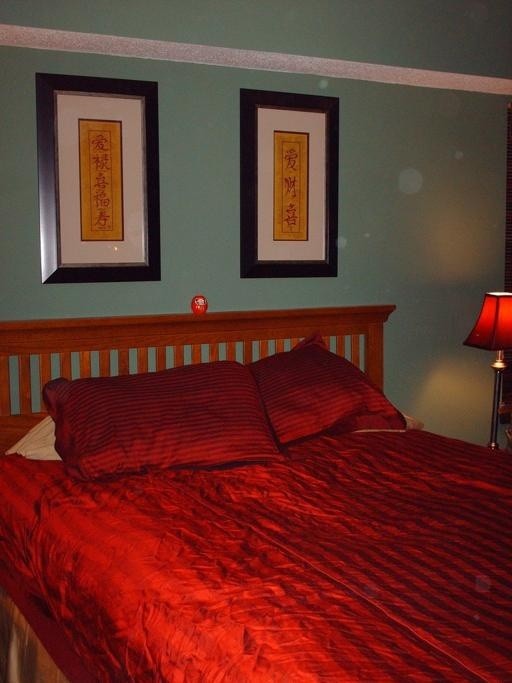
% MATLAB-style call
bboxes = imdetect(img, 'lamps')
[460,288,511,450]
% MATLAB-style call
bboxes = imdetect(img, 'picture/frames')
[33,67,164,284]
[237,84,342,280]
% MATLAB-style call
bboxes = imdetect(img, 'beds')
[0,300,510,681]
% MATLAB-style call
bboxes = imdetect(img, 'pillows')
[242,326,408,447]
[40,358,290,483]
